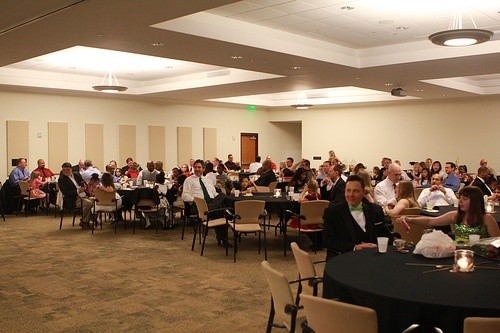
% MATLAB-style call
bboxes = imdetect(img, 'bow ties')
[349,205,363,212]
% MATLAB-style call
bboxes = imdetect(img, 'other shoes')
[39,206,48,211]
[143,223,153,230]
[79,219,93,229]
[309,244,323,252]
[218,239,232,248]
[24,207,35,215]
[110,216,123,225]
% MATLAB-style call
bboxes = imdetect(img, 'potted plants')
[453,224,479,246]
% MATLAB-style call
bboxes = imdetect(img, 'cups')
[469,235,480,247]
[274,188,281,197]
[454,250,474,272]
[377,238,389,253]
[485,202,495,212]
[427,202,433,210]
[235,190,239,197]
[290,187,294,194]
[396,240,405,251]
[123,180,154,189]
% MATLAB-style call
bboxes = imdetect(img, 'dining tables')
[323,244,500,333]
[224,192,295,250]
[114,184,156,231]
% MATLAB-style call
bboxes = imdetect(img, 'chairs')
[0,173,500,333]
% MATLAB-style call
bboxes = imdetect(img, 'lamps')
[93,69,129,94]
[428,4,494,47]
[290,91,314,109]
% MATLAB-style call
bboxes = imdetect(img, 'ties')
[23,171,25,176]
[199,178,211,203]
[393,183,396,195]
[42,170,47,177]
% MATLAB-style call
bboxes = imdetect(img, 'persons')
[385,180,420,217]
[10,158,55,215]
[417,173,459,211]
[322,175,407,265]
[182,160,232,248]
[203,154,241,186]
[249,156,263,173]
[374,163,402,205]
[395,186,500,240]
[341,157,393,203]
[250,160,277,186]
[289,180,321,231]
[58,162,93,230]
[320,164,345,201]
[266,156,276,171]
[72,157,195,229]
[279,150,345,193]
[231,175,258,194]
[395,158,500,202]
